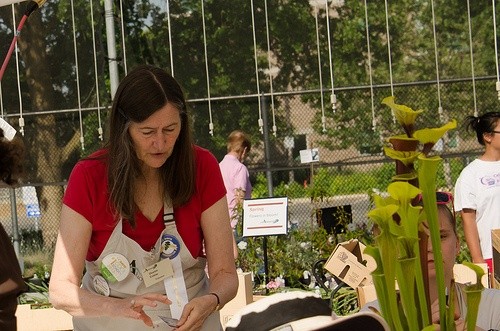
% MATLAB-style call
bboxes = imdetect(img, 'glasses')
[411,192,456,228]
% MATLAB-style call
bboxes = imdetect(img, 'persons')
[395,197,494,327]
[51,66,239,331]
[453,109,500,294]
[209,129,266,238]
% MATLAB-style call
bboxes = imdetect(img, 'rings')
[129,295,140,310]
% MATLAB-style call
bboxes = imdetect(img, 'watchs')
[208,292,221,311]
